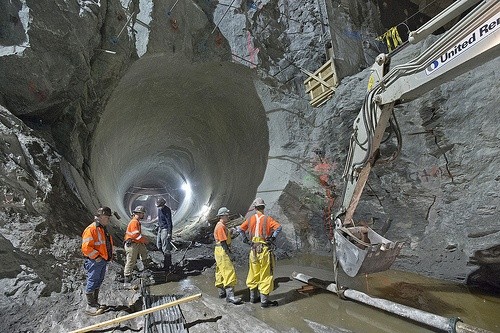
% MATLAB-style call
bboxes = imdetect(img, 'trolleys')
[207,209,246,238]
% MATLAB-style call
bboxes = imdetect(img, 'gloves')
[266,236,276,246]
[239,230,250,246]
[167,233,172,241]
[221,240,236,262]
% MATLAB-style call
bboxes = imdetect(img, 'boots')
[260,293,279,308]
[142,259,153,273]
[249,288,260,303]
[225,286,242,304]
[163,253,172,272]
[85,292,103,316]
[217,287,225,298]
[159,247,165,263]
[124,275,138,290]
[93,289,106,310]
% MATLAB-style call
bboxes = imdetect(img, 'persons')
[155,197,172,273]
[214,207,242,304]
[124,205,149,290]
[81,207,113,316]
[238,198,282,308]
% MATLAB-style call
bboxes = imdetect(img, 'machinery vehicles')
[325,0,500,278]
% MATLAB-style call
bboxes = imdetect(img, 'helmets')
[97,207,112,216]
[155,196,166,206]
[216,207,230,216]
[252,198,266,207]
[132,206,147,213]
